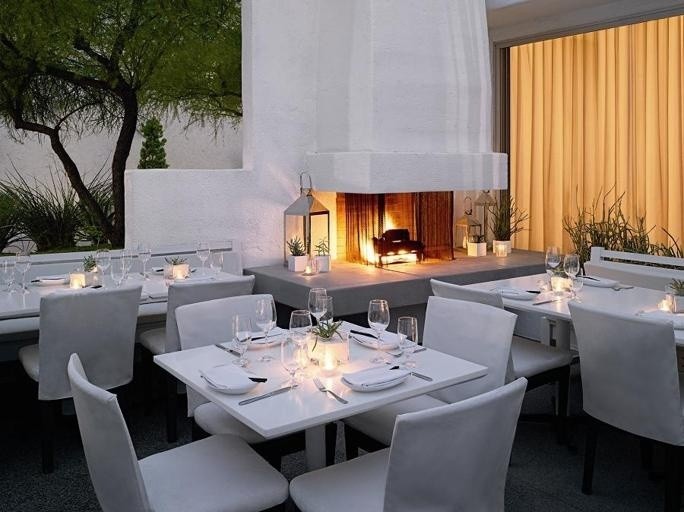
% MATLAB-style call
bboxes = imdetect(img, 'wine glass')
[91,242,223,289]
[1,252,32,297]
[232,287,334,392]
[546,247,584,303]
[368,300,419,369]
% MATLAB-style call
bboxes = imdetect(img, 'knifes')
[215,344,240,356]
[387,361,432,381]
[532,299,558,305]
[238,385,298,405]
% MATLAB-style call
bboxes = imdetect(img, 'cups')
[70,278,82,289]
[665,283,674,295]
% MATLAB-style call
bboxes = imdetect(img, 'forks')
[612,285,634,291]
[312,377,347,404]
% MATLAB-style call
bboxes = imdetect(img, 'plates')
[578,274,619,288]
[342,365,411,392]
[200,369,259,395]
[638,310,684,330]
[352,335,398,350]
[36,277,70,285]
[490,286,539,300]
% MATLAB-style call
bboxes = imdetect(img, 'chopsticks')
[249,377,267,382]
